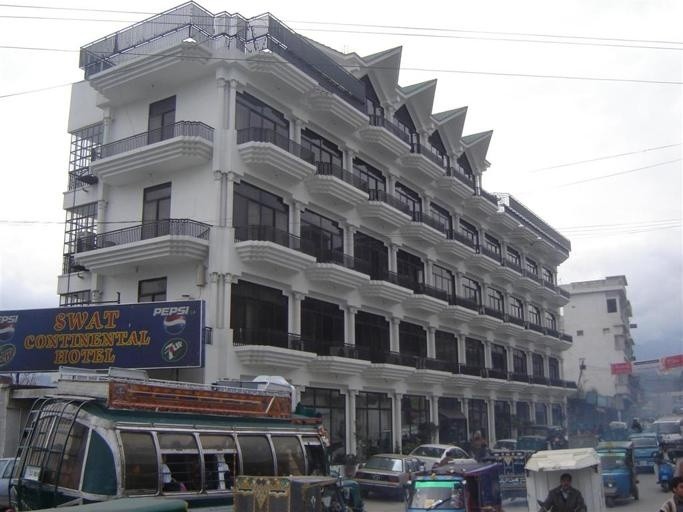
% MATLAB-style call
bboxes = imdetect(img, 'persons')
[535,418,683,511]
[161,455,186,491]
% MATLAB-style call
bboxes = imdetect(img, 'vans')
[625,433,662,470]
[493,439,516,451]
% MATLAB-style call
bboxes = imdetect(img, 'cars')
[354,454,425,503]
[407,444,478,471]
[0,457,20,509]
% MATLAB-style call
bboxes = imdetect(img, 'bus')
[8,376,333,511]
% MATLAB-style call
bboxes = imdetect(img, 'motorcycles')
[654,459,672,492]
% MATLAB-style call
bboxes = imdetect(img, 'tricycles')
[523,447,606,512]
[595,442,638,508]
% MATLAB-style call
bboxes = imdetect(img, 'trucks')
[485,423,562,499]
[405,464,502,512]
[232,474,363,512]
[649,416,682,453]
